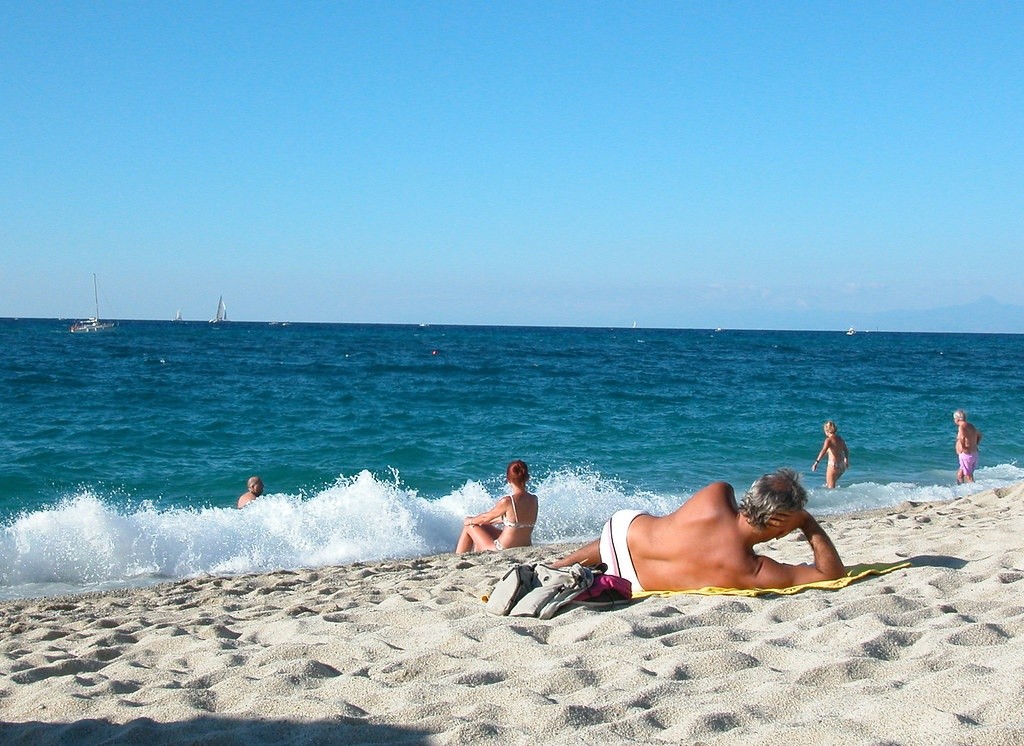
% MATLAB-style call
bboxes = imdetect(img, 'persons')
[953,410,981,485]
[454,460,538,553]
[235,477,266,510]
[811,421,849,490]
[546,468,846,595]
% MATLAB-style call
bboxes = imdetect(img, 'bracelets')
[815,460,819,463]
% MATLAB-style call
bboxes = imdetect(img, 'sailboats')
[209,295,229,324]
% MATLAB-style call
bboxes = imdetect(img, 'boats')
[70,272,115,333]
